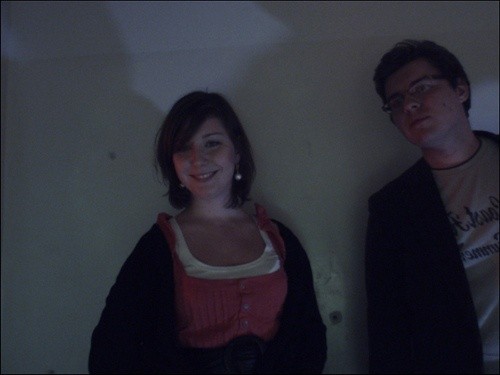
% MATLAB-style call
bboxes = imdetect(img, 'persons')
[88,90,329,375]
[364,40,499,375]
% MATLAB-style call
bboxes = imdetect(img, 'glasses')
[382,74,449,115]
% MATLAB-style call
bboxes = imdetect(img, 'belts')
[179,333,276,375]
